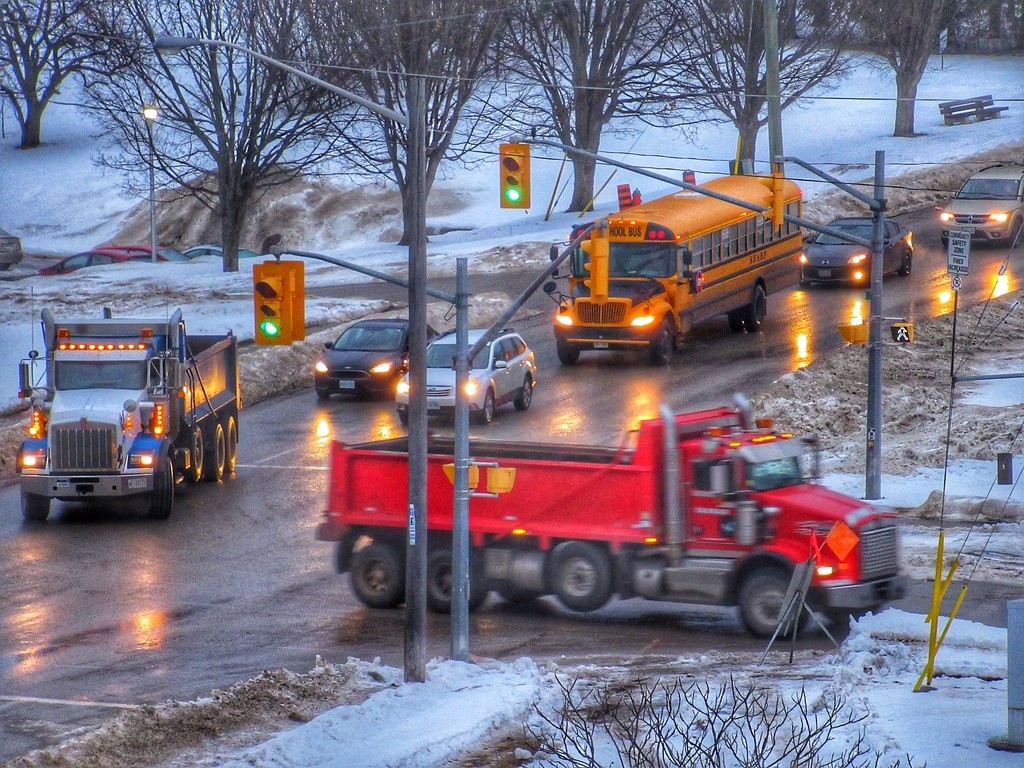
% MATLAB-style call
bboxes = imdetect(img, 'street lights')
[149,35,428,686]
[143,107,158,261]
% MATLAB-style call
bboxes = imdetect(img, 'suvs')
[939,162,1024,251]
[396,328,537,427]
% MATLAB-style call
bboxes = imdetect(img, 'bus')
[542,161,802,367]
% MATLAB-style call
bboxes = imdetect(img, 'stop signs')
[696,271,704,292]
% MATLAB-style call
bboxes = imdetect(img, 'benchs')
[939,95,1009,126]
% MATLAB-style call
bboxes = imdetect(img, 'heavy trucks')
[315,392,910,642]
[17,307,242,523]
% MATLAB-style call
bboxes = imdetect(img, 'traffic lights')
[253,263,293,346]
[892,323,914,343]
[498,143,530,210]
[757,172,784,227]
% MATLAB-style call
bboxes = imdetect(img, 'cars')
[314,318,439,400]
[800,217,915,291]
[0,227,24,270]
[38,242,259,277]
